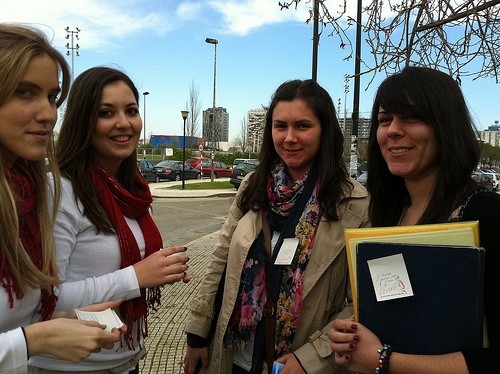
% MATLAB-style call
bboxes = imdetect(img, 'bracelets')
[376,344,392,374]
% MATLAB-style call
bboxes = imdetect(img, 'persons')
[0,23,191,374]
[184,80,374,374]
[327,67,500,374]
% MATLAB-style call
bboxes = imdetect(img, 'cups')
[159,247,188,273]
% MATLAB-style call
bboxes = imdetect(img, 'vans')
[185,156,211,168]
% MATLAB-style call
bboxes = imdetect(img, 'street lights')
[142,92,149,159]
[180,110,189,188]
[205,37,218,182]
[65,25,81,81]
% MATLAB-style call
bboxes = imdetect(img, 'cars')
[154,160,202,181]
[193,161,233,178]
[470,166,500,194]
[228,158,258,190]
[355,161,368,187]
[136,158,160,183]
